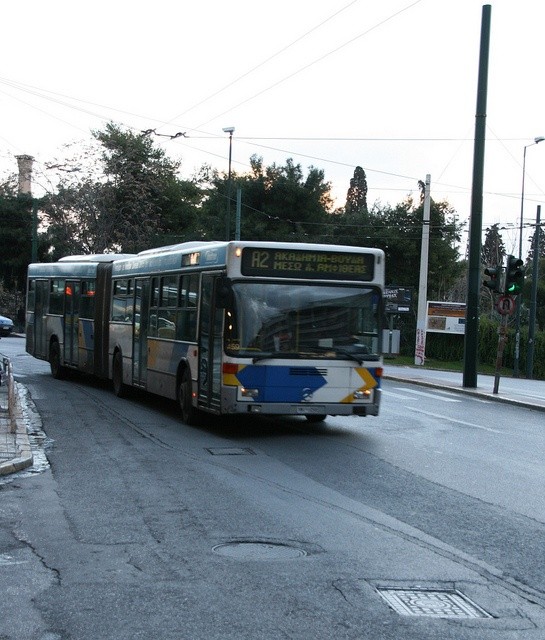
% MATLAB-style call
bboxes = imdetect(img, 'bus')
[24,239,386,429]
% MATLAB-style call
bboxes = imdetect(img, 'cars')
[0,314,15,338]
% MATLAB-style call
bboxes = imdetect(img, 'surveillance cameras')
[223,126,236,135]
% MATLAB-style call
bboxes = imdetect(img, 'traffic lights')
[503,255,523,295]
[483,266,501,293]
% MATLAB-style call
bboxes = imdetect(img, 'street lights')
[519,137,545,259]
[223,127,235,239]
